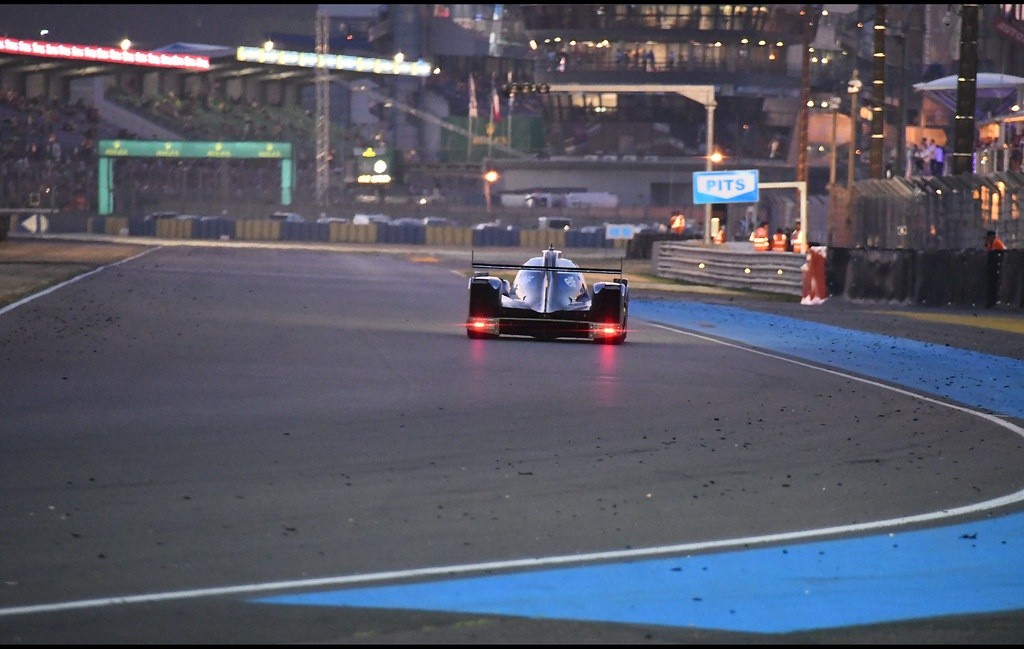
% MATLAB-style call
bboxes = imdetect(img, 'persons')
[669,209,687,235]
[751,222,769,251]
[712,225,727,245]
[769,227,788,251]
[907,133,1024,174]
[524,3,728,84]
[0,83,372,218]
[790,218,809,253]
[984,231,1006,249]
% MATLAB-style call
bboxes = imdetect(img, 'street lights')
[481,159,499,213]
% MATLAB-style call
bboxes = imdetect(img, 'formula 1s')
[466,243,630,345]
